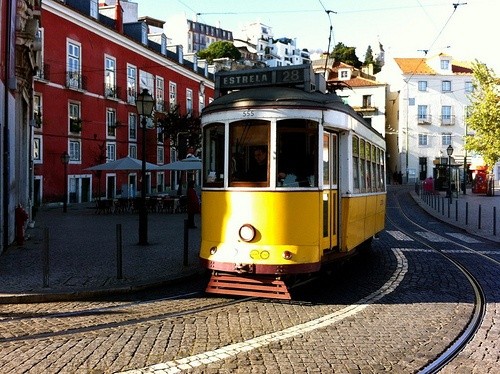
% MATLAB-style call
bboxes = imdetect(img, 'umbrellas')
[83,154,161,211]
[150,156,202,194]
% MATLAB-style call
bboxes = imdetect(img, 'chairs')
[96,195,187,216]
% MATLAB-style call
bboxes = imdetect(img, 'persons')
[186,180,199,229]
[392,171,403,185]
[231,147,286,182]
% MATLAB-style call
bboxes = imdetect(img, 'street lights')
[133,87,156,247]
[446,144,454,198]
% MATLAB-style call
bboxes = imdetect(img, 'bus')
[175,60,390,301]
